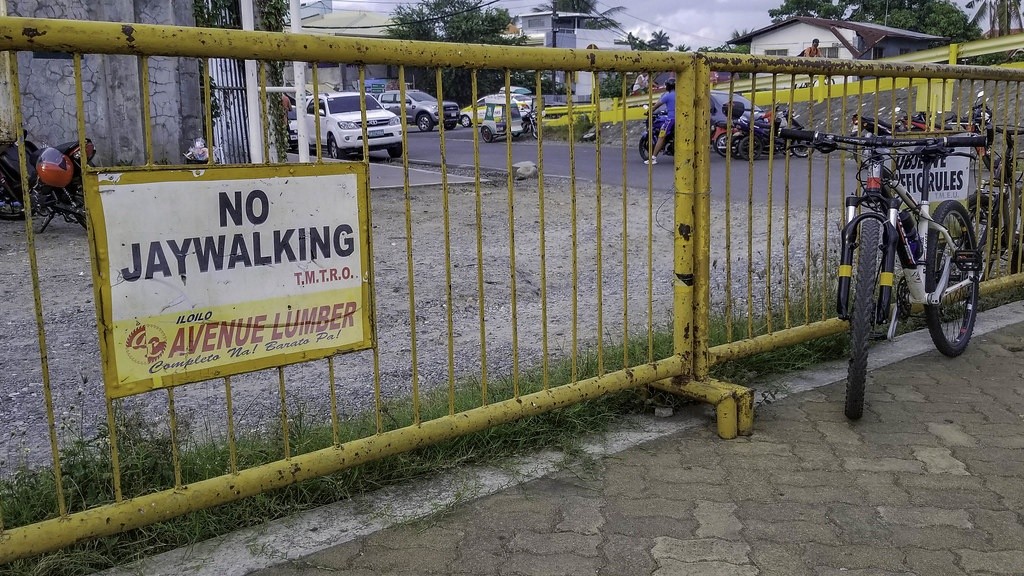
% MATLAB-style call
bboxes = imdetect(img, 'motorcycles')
[0,128,99,234]
[479,95,537,143]
[849,90,995,171]
[639,99,815,161]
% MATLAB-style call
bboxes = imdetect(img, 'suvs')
[306,92,403,159]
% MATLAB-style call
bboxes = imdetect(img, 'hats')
[814,39,819,43]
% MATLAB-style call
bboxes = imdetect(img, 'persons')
[282,94,293,127]
[633,71,659,92]
[804,39,823,57]
[643,78,675,165]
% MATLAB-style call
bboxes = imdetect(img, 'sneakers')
[644,158,658,165]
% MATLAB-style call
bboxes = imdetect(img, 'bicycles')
[937,123,1024,279]
[795,75,834,87]
[775,126,982,418]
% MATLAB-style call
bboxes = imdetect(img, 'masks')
[813,44,818,47]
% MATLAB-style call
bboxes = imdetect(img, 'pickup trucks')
[372,90,461,132]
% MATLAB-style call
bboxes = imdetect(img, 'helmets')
[665,78,675,91]
[36,147,73,186]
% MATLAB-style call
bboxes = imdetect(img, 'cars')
[287,104,298,149]
[458,94,547,127]
[659,92,769,135]
[631,68,740,89]
